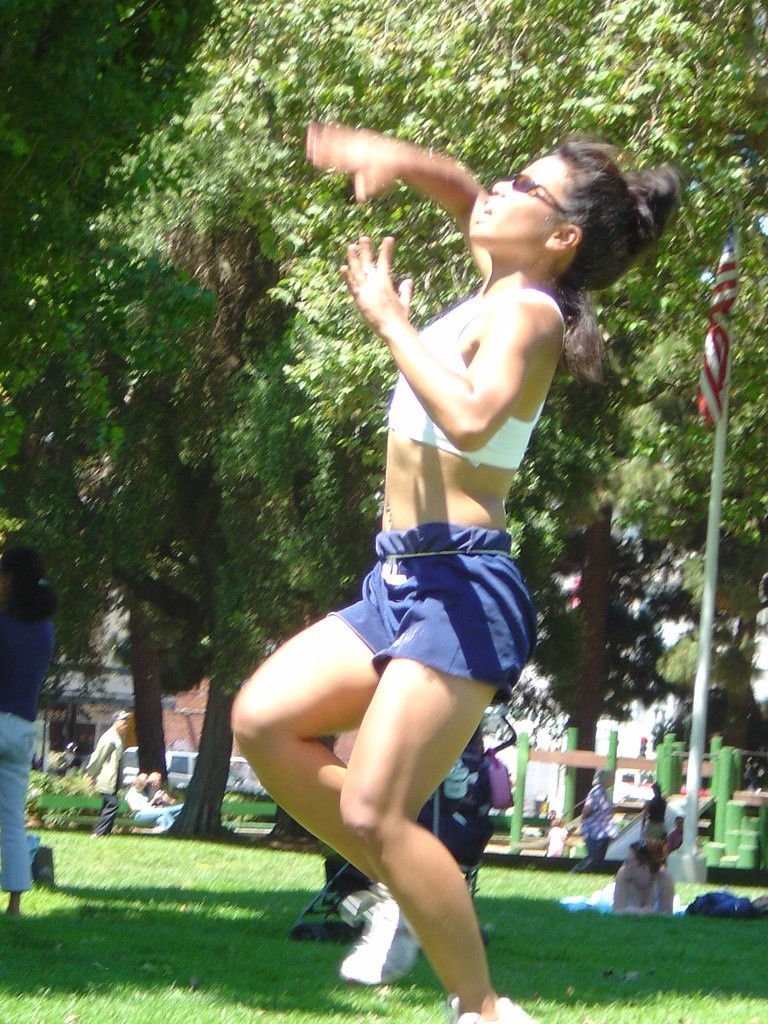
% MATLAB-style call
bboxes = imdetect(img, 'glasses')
[508,172,578,219]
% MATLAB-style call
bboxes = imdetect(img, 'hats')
[112,710,133,722]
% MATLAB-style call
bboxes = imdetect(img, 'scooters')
[47,750,83,780]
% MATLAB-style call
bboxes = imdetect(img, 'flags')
[695,240,738,426]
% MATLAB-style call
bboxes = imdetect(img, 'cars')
[121,747,200,791]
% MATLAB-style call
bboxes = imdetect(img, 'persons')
[0,544,58,915]
[59,740,81,776]
[230,121,683,1024]
[545,818,568,858]
[84,710,133,836]
[570,771,684,914]
[125,772,184,828]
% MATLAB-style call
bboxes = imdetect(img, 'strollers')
[284,716,519,944]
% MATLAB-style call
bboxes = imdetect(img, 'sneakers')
[338,882,417,985]
[446,993,531,1024]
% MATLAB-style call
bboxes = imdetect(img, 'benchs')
[219,802,279,828]
[39,796,145,824]
